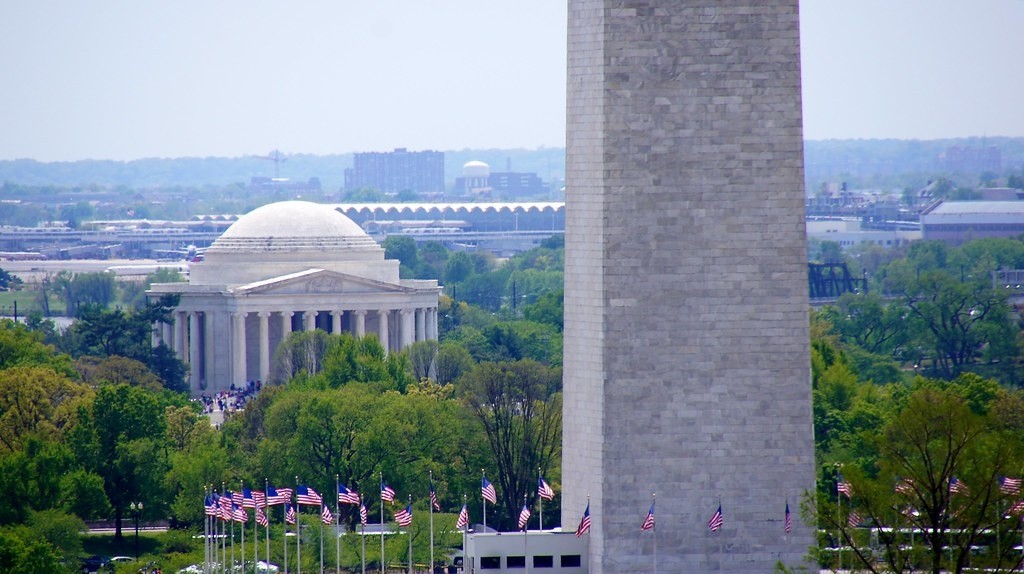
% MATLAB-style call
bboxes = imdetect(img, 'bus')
[871,527,1024,560]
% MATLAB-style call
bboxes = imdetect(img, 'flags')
[321,503,334,525]
[381,481,395,501]
[429,479,440,511]
[296,485,322,506]
[481,477,497,504]
[836,474,853,497]
[575,504,591,537]
[360,505,367,527]
[338,482,361,505]
[784,504,791,533]
[641,504,655,531]
[456,504,469,529]
[267,486,293,505]
[847,510,864,528]
[949,476,970,497]
[394,504,412,527]
[538,476,555,501]
[997,475,1024,515]
[708,505,723,532]
[895,475,916,496]
[204,488,268,527]
[518,505,531,528]
[286,503,295,525]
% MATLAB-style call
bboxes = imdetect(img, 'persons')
[198,379,262,431]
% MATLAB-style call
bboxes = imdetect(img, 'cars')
[65,554,135,574]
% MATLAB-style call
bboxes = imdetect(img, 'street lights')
[130,502,145,561]
